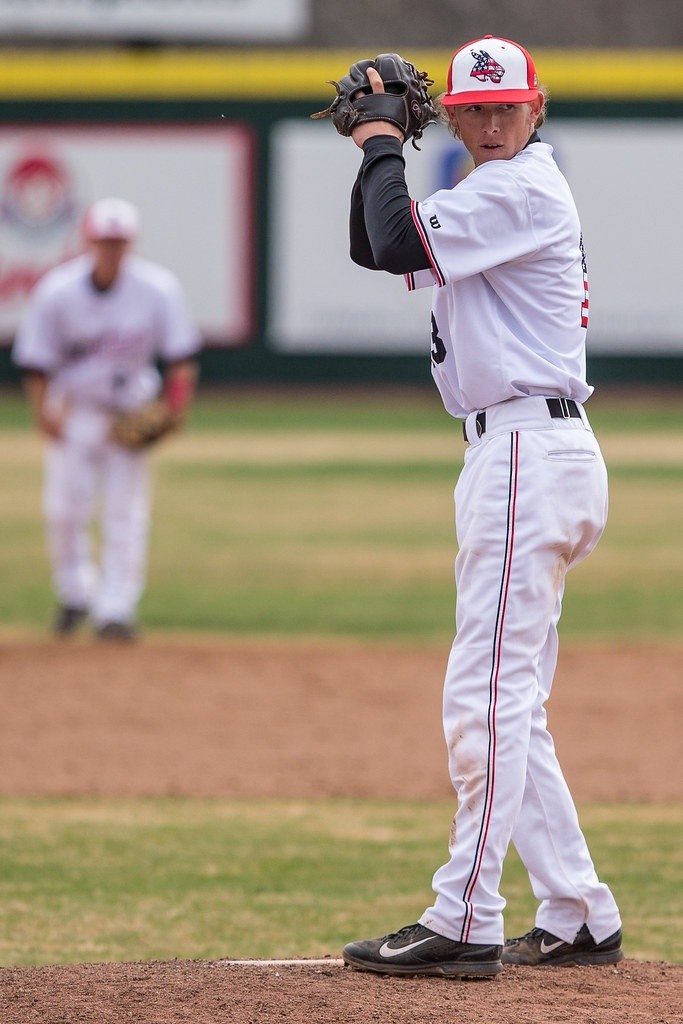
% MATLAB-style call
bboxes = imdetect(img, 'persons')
[14,191,204,652]
[307,34,626,982]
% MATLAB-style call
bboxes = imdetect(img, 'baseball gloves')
[309,52,438,152]
[110,403,177,452]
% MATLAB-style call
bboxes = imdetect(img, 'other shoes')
[99,623,136,652]
[45,608,88,655]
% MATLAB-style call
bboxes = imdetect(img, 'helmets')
[83,198,139,240]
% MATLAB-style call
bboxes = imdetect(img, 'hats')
[443,36,539,106]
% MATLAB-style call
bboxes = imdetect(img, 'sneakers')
[502,923,622,966]
[341,923,503,980]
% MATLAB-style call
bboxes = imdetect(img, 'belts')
[462,398,582,442]
